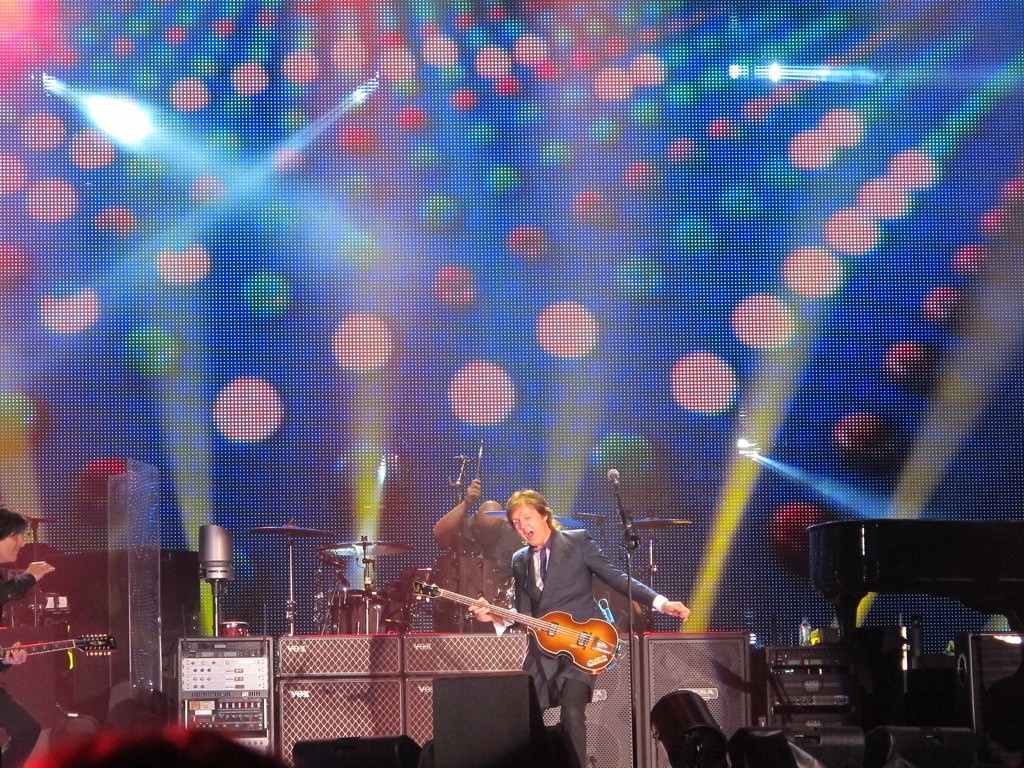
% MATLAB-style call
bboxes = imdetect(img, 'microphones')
[608,469,620,488]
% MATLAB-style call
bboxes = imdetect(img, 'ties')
[539,548,547,585]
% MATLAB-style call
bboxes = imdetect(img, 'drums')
[432,554,497,619]
[322,588,391,635]
[384,583,417,628]
[401,567,432,614]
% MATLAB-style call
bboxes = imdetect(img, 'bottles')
[798,617,811,646]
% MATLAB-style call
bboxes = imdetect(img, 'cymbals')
[614,518,695,529]
[248,526,334,538]
[484,511,585,527]
[317,543,416,558]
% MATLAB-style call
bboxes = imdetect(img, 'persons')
[0,508,56,768]
[433,480,529,605]
[468,490,690,768]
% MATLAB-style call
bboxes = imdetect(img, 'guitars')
[1,630,117,665]
[412,579,621,674]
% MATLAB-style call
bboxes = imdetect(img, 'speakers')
[727,726,865,768]
[278,678,404,768]
[866,725,977,768]
[433,673,550,768]
[293,735,422,768]
[404,634,527,675]
[276,634,403,676]
[542,632,642,768]
[641,634,752,768]
[405,678,433,747]
